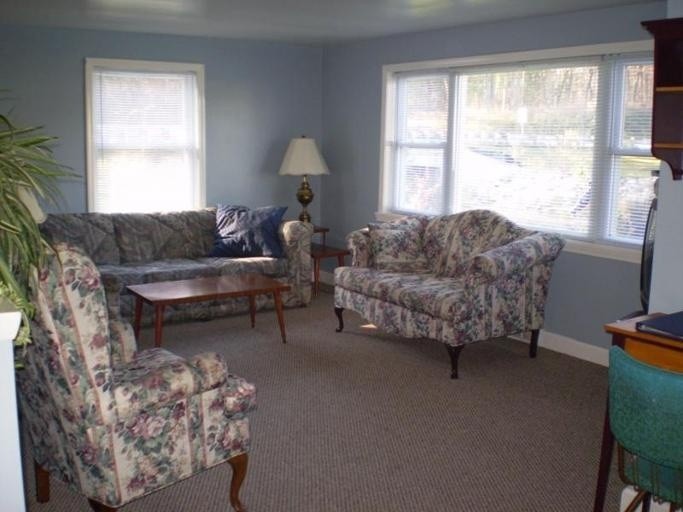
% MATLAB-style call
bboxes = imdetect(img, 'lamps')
[280,135,330,223]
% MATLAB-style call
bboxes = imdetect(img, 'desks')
[313,229,348,298]
[592,314,682,511]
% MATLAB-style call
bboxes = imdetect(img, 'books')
[636,310,683,340]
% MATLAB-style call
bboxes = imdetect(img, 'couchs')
[20,235,257,511]
[332,209,562,381]
[41,209,314,329]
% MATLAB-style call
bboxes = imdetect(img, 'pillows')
[208,204,288,260]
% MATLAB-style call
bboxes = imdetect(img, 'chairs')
[609,345,683,512]
